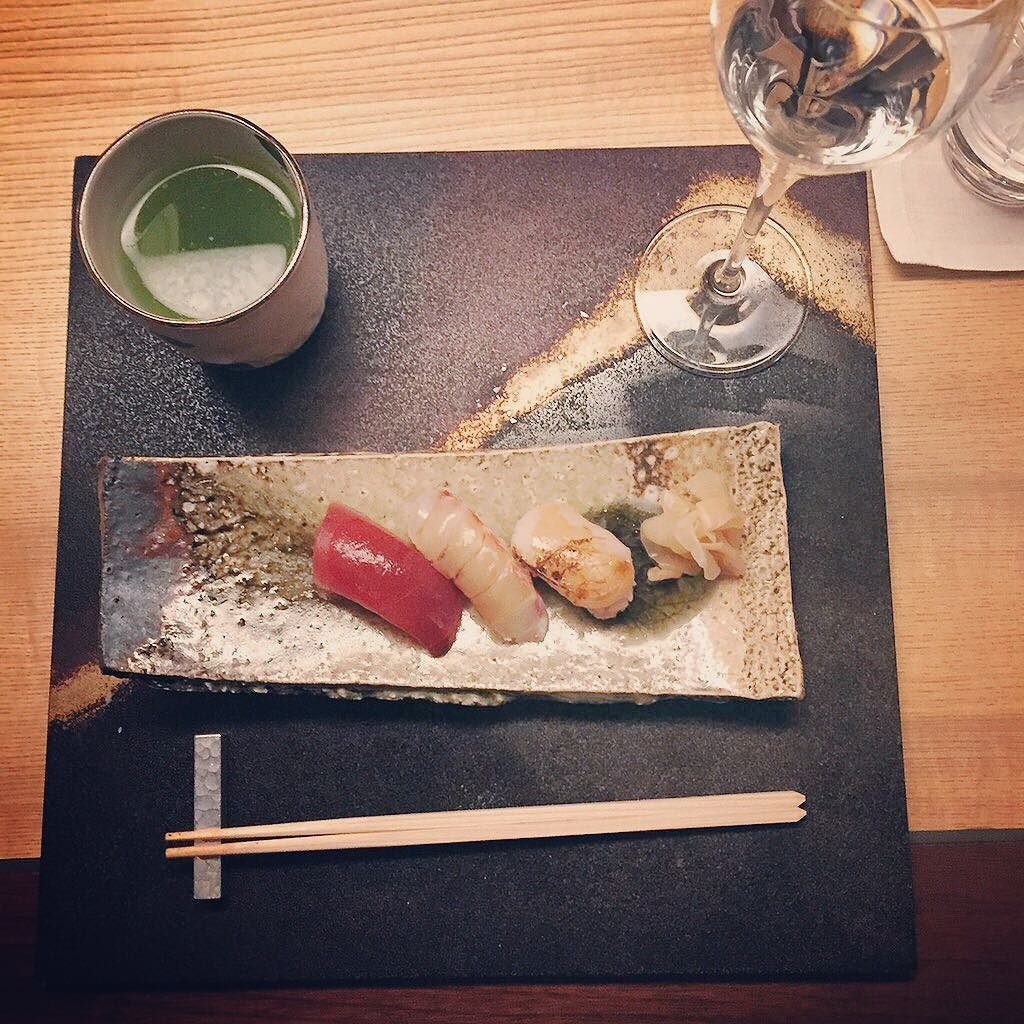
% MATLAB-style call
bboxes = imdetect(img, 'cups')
[75,105,329,376]
[939,18,1024,214]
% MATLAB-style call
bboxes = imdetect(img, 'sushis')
[311,502,637,659]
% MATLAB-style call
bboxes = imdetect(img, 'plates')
[96,421,806,704]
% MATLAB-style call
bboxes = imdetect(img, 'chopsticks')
[164,790,808,859]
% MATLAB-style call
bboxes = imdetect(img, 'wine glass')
[636,0,1024,377]
